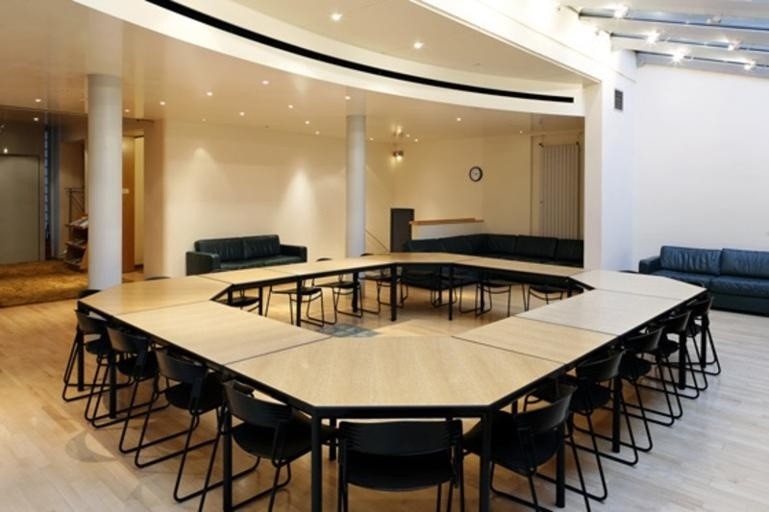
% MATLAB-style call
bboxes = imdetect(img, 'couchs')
[395,231,585,293]
[186,234,308,275]
[636,245,768,317]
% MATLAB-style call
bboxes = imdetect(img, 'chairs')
[61,253,722,511]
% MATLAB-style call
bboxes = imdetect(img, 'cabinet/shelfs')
[64,187,88,272]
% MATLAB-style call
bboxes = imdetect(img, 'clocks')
[469,167,483,182]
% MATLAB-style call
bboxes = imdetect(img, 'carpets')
[0,258,135,309]
